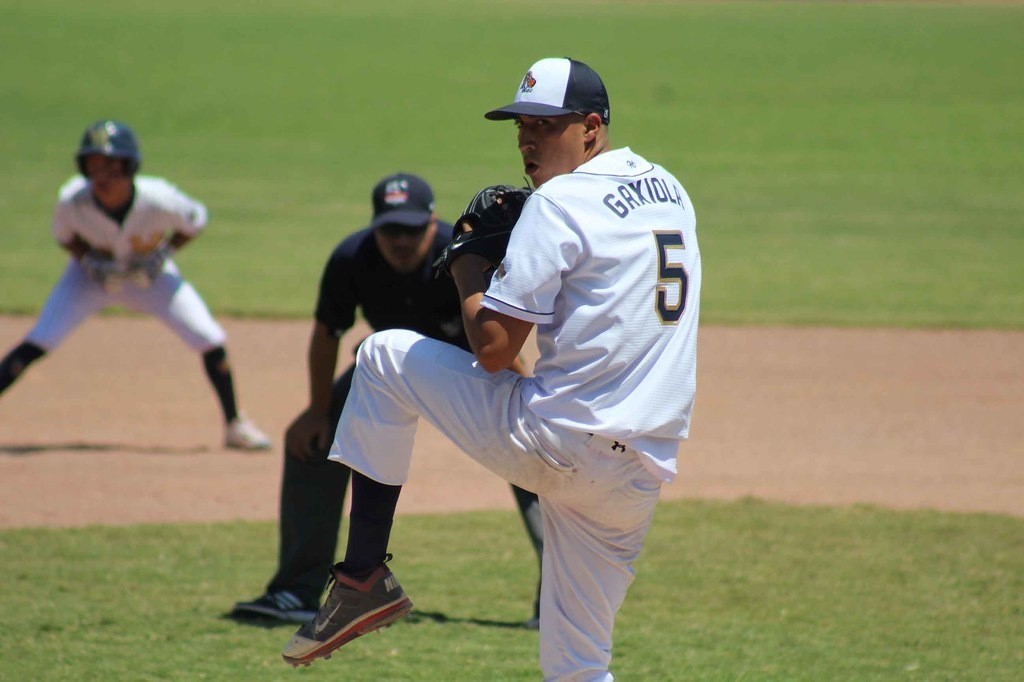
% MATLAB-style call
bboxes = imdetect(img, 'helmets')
[76,119,140,173]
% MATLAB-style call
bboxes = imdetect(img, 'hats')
[372,173,435,227]
[483,56,611,126]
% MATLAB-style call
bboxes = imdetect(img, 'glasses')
[380,223,428,239]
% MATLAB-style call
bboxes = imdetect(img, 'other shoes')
[225,420,272,451]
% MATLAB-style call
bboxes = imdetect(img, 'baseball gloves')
[432,184,536,280]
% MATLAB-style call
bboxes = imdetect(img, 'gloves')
[82,252,125,296]
[136,241,173,288]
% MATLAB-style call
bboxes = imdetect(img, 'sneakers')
[232,580,321,628]
[283,554,414,669]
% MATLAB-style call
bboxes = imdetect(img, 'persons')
[281,55,702,681]
[233,172,544,629]
[0,118,271,453]
